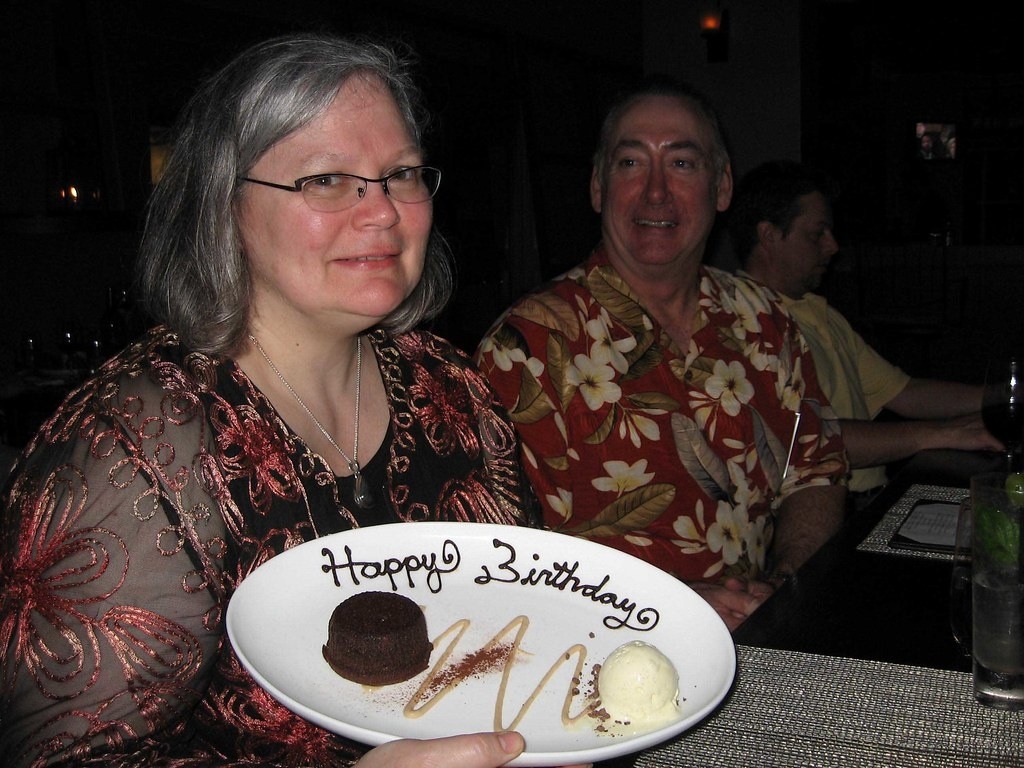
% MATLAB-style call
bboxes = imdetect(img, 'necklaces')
[243,328,374,508]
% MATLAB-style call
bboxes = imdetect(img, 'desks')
[591,449,1024,767]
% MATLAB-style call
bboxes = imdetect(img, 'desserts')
[599,642,680,721]
[326,591,430,686]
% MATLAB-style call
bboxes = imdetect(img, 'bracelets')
[770,570,790,585]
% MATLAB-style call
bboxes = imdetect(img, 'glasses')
[242,165,442,213]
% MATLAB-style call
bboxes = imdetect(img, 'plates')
[226,520,737,765]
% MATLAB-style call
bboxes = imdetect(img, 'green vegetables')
[974,497,1020,574]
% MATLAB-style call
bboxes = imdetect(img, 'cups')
[968,471,1024,713]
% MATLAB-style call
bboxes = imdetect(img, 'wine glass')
[980,353,1024,491]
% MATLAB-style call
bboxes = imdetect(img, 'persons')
[471,90,850,632]
[917,124,955,161]
[722,171,1007,505]
[1,34,542,768]
